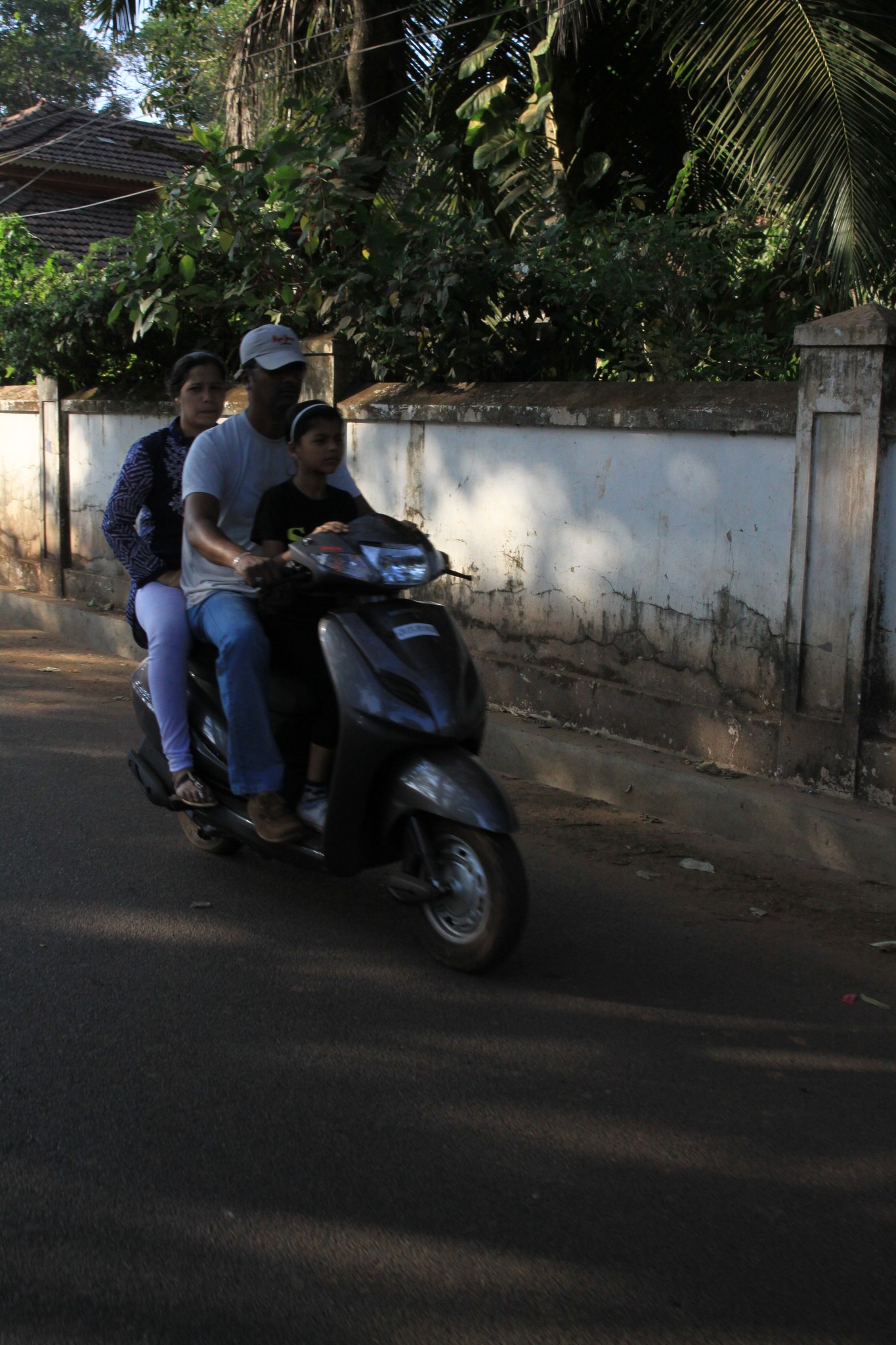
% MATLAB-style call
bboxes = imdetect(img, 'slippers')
[171,768,217,806]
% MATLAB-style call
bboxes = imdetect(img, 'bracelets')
[233,552,252,567]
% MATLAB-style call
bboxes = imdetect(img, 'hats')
[234,324,306,379]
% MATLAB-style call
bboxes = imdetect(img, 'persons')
[101,352,226,807]
[180,325,371,842]
[249,399,417,835]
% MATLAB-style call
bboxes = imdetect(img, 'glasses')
[243,366,309,381]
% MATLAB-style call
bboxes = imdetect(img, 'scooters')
[122,512,531,974]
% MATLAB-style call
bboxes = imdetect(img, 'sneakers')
[295,798,329,834]
[245,792,304,843]
